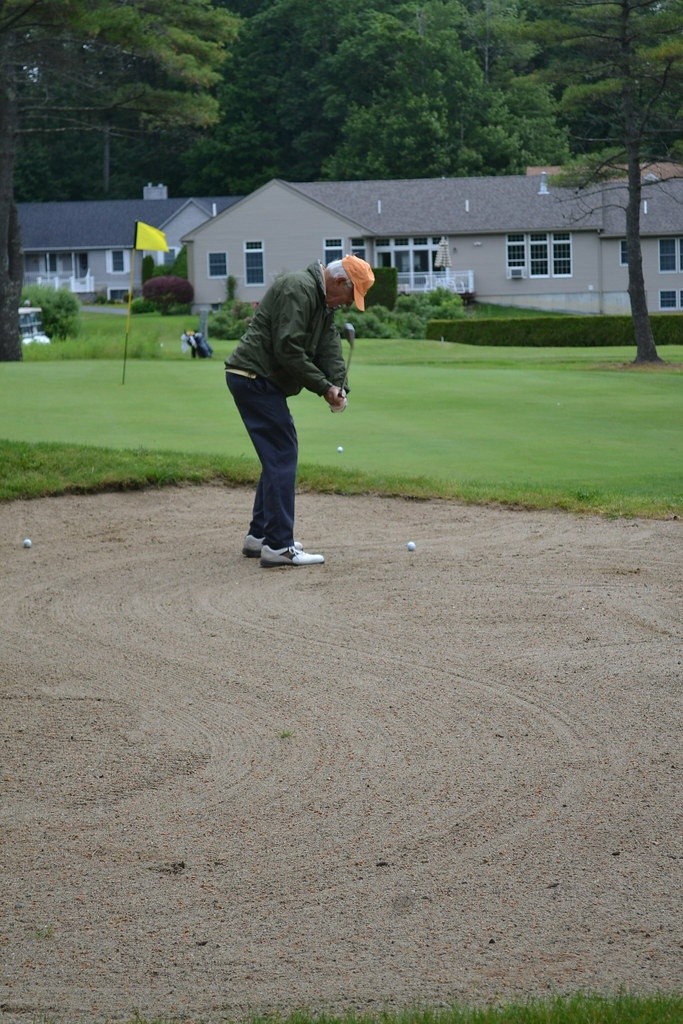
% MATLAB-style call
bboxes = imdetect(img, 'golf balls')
[406,542,416,551]
[22,539,32,548]
[337,447,343,453]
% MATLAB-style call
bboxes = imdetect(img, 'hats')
[342,254,374,313]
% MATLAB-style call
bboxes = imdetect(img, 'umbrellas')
[434,235,452,277]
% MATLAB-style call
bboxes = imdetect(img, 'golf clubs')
[330,323,357,413]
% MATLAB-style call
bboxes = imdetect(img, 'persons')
[184,329,213,358]
[224,254,375,567]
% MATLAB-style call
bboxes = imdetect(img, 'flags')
[135,221,170,252]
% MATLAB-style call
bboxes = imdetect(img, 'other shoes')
[259,542,325,567]
[241,533,303,558]
